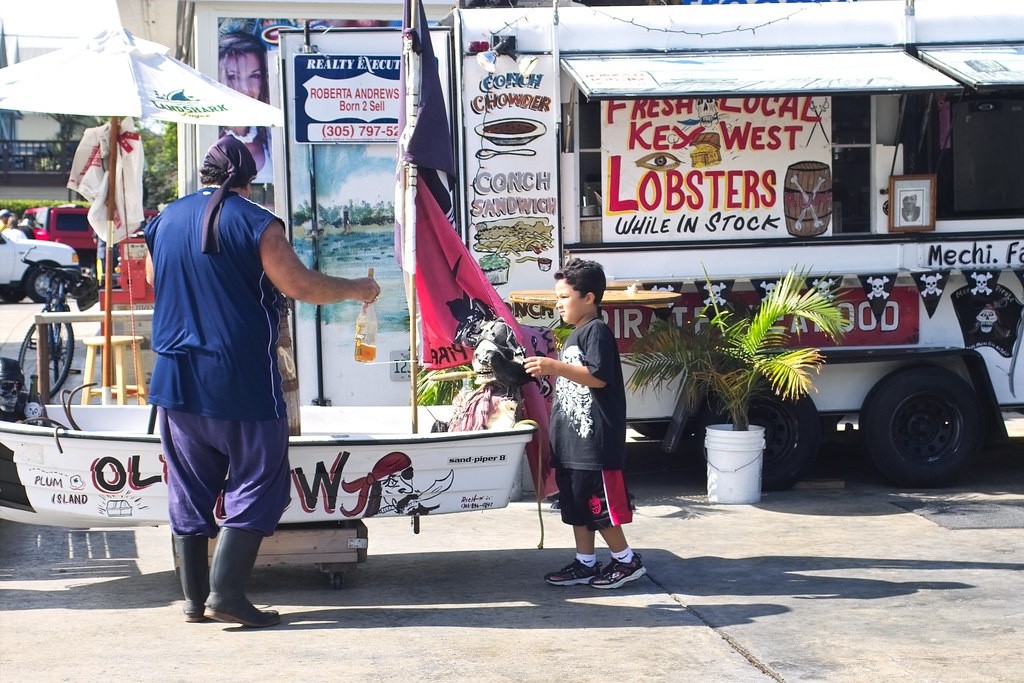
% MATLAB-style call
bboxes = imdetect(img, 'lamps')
[475,35,538,77]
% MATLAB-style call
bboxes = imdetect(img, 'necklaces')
[224,125,257,144]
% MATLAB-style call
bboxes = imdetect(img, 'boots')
[176,535,210,622]
[203,527,280,628]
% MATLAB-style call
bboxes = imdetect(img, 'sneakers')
[589,552,647,589]
[544,558,602,586]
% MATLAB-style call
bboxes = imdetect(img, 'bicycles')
[14,244,99,406]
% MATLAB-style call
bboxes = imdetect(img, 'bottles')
[355,268,377,363]
[24,375,42,426]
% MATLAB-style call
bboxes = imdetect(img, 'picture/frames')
[888,174,936,233]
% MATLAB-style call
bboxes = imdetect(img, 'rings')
[374,295,379,300]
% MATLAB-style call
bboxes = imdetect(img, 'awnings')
[561,47,963,180]
[919,46,1024,182]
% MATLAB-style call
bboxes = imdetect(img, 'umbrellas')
[0,25,284,405]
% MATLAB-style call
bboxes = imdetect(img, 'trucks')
[552,41,1024,494]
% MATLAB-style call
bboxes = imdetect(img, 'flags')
[395,0,562,498]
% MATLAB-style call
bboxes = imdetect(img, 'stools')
[80,336,146,405]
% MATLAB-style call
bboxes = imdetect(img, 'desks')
[511,291,681,321]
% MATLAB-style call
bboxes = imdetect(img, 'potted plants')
[419,326,568,501]
[635,260,853,506]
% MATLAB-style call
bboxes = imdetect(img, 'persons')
[0,208,41,240]
[522,258,648,588]
[142,135,380,627]
[92,231,120,285]
[218,30,273,184]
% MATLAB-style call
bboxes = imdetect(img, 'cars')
[0,231,80,305]
[23,206,97,264]
